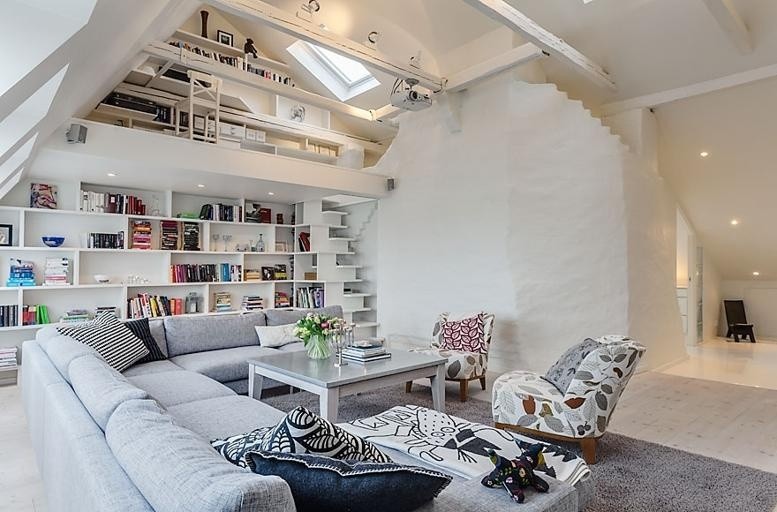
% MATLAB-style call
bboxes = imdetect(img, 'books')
[79,189,147,215]
[201,203,243,223]
[1,304,51,326]
[60,307,116,323]
[0,347,18,368]
[130,219,200,250]
[6,257,72,286]
[275,286,324,309]
[212,292,232,313]
[240,296,264,311]
[127,292,184,319]
[336,337,391,364]
[298,232,311,252]
[178,38,289,86]
[170,263,241,283]
[245,264,288,282]
[245,202,271,224]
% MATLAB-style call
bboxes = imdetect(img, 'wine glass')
[223,235,229,252]
[213,233,219,252]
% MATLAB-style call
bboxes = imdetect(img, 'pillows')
[209,405,453,512]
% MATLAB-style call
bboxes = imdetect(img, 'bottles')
[256,234,265,252]
[185,292,200,313]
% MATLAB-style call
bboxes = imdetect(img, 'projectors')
[390,87,432,112]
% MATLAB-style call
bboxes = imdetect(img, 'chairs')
[405,312,647,464]
[724,299,757,343]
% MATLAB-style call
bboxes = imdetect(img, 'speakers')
[388,179,394,191]
[67,124,87,144]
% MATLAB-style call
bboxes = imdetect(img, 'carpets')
[217,381,777,512]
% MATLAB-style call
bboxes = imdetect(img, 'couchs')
[19,305,596,512]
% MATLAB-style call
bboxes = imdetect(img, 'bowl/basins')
[41,236,65,247]
[95,275,113,284]
